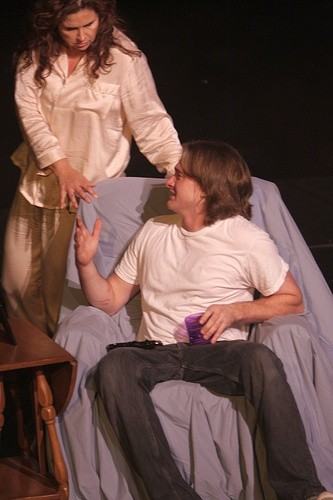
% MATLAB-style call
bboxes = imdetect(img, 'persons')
[3,1,185,341]
[72,141,333,499]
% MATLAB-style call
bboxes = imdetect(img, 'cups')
[185,313,211,347]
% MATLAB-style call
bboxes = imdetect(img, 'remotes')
[108,339,163,349]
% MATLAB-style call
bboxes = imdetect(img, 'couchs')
[40,176,331,500]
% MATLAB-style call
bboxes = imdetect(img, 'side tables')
[1,314,78,500]
